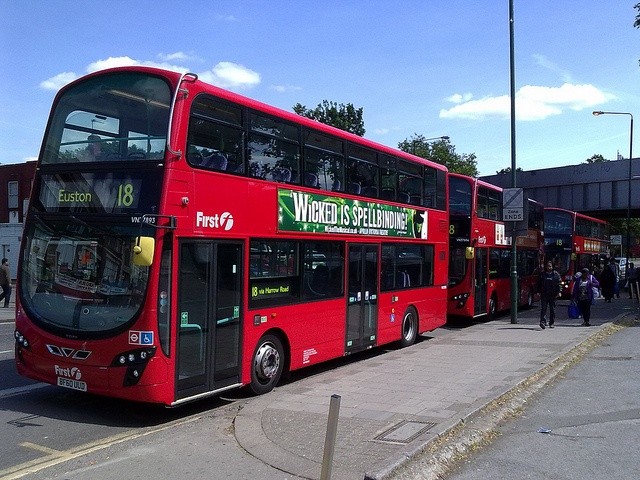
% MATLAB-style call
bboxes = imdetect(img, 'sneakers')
[540,322,545,329]
[549,322,554,328]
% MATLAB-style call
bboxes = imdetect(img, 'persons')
[625,262,639,300]
[537,261,563,330]
[608,258,621,299]
[0,258,13,309]
[572,267,599,327]
[599,264,616,303]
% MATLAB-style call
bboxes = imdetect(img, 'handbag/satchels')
[591,275,601,299]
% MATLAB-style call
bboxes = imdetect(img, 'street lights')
[592,110,634,292]
[409,133,451,167]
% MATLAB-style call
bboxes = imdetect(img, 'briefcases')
[568,300,582,318]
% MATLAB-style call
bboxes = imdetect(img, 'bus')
[546,207,611,296]
[14,66,448,415]
[450,173,543,328]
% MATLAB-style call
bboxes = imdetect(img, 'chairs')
[351,183,362,196]
[423,196,433,208]
[270,166,292,183]
[198,153,228,170]
[362,186,379,198]
[410,195,423,204]
[396,191,412,204]
[329,177,341,192]
[305,172,319,187]
[382,187,396,201]
